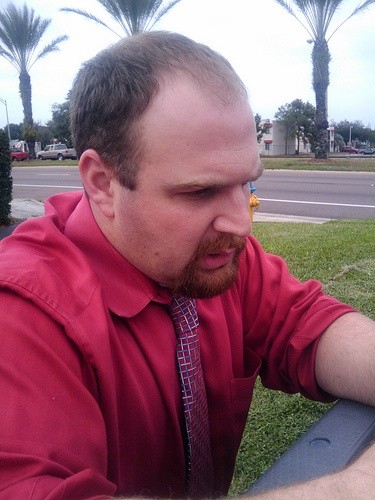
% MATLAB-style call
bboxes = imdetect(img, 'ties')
[168,293,217,500]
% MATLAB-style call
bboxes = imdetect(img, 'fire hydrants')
[249,183,260,224]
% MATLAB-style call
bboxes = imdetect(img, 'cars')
[10,147,30,161]
[359,146,375,155]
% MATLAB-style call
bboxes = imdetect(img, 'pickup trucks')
[35,144,78,160]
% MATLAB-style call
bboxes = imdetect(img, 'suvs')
[339,146,360,154]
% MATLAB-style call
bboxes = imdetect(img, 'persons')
[0,31,375,500]
